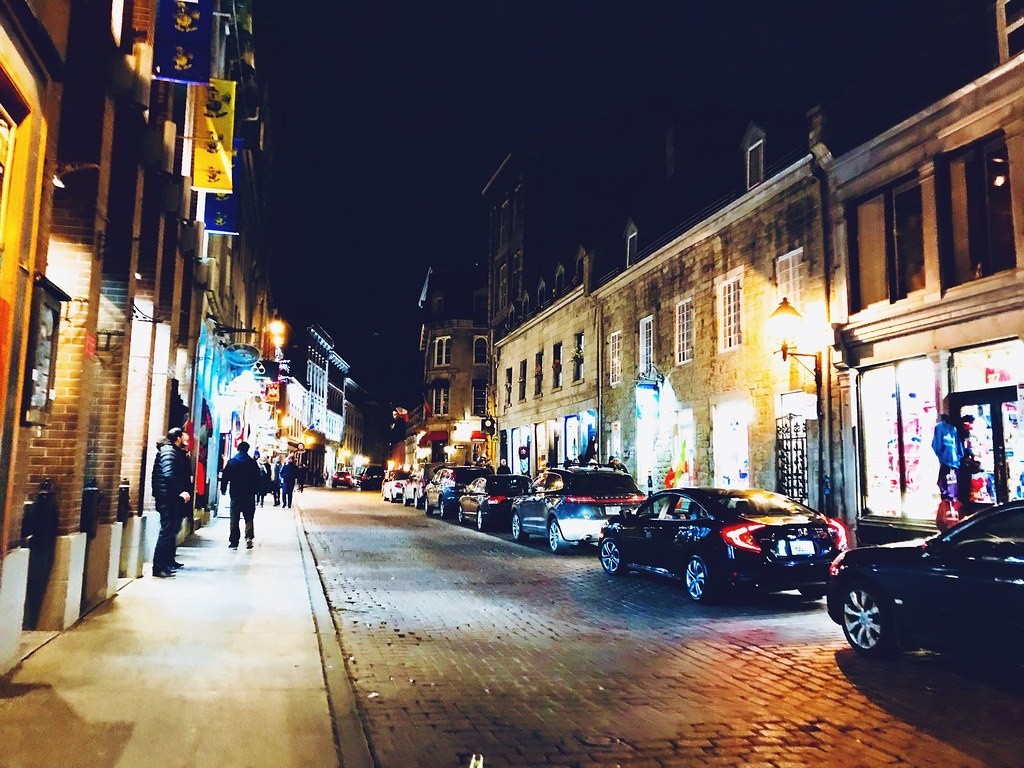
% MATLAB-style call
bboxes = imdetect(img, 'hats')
[613,455,621,462]
[261,452,267,458]
[276,456,281,461]
[609,456,614,460]
[168,427,182,441]
[253,450,260,456]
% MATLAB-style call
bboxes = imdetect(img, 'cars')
[354,474,361,486]
[456,474,534,531]
[381,469,410,503]
[827,499,1024,660]
[597,488,848,601]
[332,471,354,489]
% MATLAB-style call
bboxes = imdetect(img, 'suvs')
[360,465,385,491]
[423,467,493,517]
[402,464,449,509]
[511,466,645,554]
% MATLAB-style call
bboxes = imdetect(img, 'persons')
[496,459,511,474]
[464,457,495,474]
[250,450,329,508]
[219,441,261,550]
[563,451,629,473]
[543,462,551,471]
[151,427,190,578]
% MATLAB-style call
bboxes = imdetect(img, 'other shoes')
[260,504,263,507]
[228,544,237,549]
[272,504,276,506]
[246,540,254,549]
[288,506,290,508]
[152,561,184,577]
[255,502,257,505]
[282,505,285,507]
